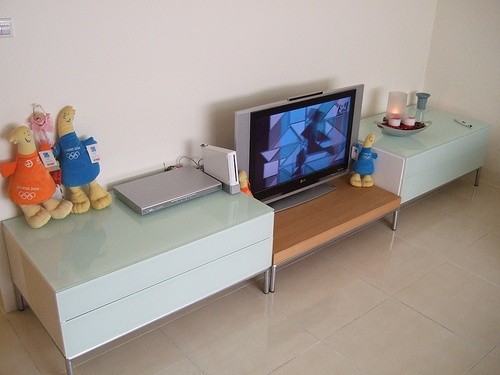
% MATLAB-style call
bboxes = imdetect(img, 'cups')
[389,116,401,126]
[402,116,416,126]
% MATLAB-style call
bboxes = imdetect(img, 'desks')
[1,191,275,375]
[270,173,401,293]
[359,104,492,231]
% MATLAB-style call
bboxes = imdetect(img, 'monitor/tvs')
[234,83,365,214]
